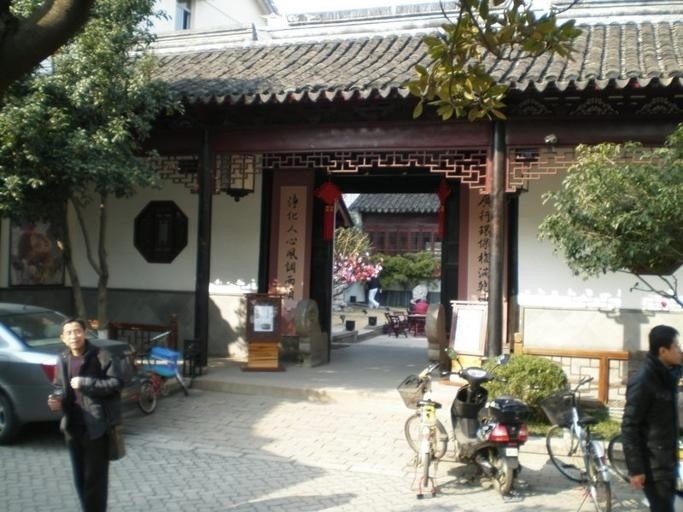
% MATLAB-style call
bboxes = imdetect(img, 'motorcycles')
[439,346,532,501]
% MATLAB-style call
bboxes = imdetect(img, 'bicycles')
[134,328,192,415]
[538,372,613,512]
[396,359,451,490]
[606,434,631,485]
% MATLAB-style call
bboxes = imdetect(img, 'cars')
[0,299,137,445]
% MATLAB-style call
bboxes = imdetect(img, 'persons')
[367,275,382,309]
[46,318,124,510]
[621,325,682,511]
[414,298,428,333]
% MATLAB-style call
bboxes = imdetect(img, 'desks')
[408,314,425,336]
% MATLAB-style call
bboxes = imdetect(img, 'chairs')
[384,312,407,337]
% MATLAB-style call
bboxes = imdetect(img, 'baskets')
[540,389,579,425]
[397,375,431,409]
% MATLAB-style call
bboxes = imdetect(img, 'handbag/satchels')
[108,424,125,461]
